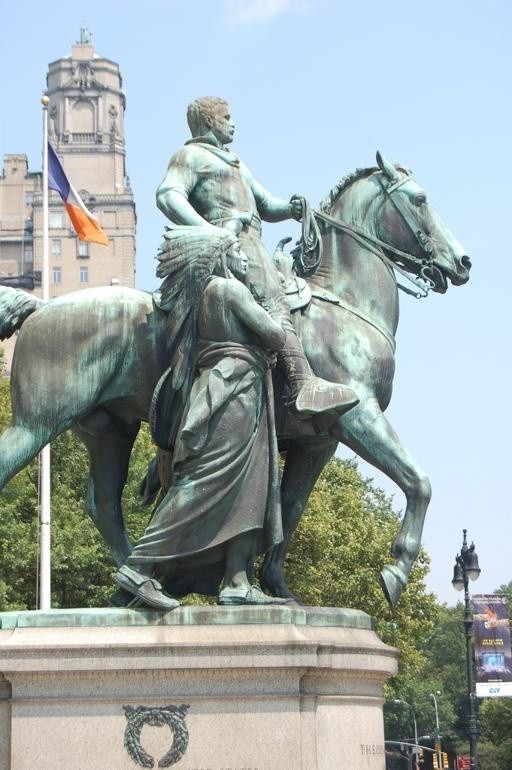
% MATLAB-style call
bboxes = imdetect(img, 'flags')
[43,109,110,248]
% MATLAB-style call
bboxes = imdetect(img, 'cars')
[386,735,457,770]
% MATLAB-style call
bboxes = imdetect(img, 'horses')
[0,150,473,612]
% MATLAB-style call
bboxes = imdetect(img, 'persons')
[109,219,293,613]
[153,92,360,439]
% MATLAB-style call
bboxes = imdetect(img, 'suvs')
[477,653,512,682]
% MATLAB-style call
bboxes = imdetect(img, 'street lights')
[394,699,419,764]
[422,693,442,768]
[452,528,481,769]
